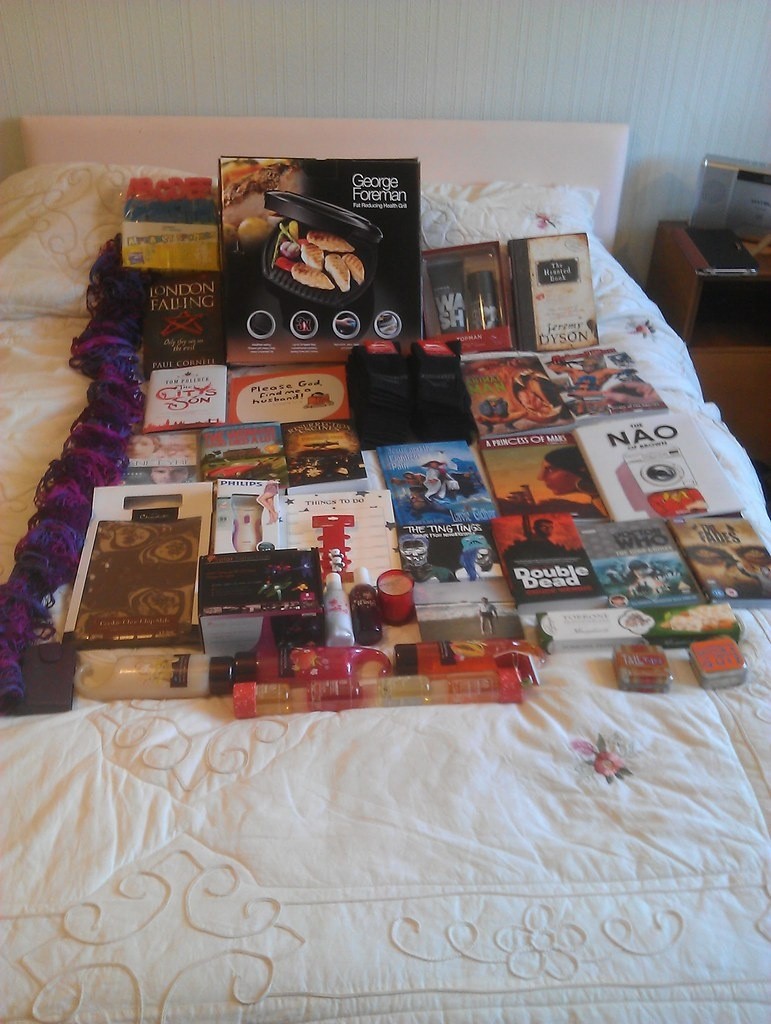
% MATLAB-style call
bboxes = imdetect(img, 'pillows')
[0,164,219,319]
[420,182,663,320]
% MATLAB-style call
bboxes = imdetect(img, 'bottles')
[348,567,381,646]
[323,573,354,647]
[75,639,546,719]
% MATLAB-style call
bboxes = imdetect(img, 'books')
[61,347,771,649]
[687,227,759,273]
[140,156,421,377]
[507,233,599,352]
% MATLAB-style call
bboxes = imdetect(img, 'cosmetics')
[323,574,355,648]
[430,258,498,335]
[349,566,383,646]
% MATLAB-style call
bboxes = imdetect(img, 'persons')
[478,595,498,636]
[531,518,553,540]
[126,434,160,460]
[736,561,771,595]
[258,482,279,524]
[151,466,188,484]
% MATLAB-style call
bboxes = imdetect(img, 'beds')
[0,116,771,1024]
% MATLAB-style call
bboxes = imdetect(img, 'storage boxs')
[218,155,421,366]
[197,548,324,658]
[420,240,518,352]
[535,602,742,656]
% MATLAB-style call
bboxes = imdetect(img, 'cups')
[377,569,414,625]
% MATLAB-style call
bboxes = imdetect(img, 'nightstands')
[646,219,771,469]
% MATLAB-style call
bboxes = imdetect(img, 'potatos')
[222,216,275,253]
